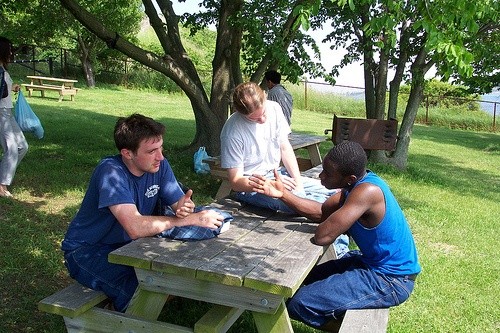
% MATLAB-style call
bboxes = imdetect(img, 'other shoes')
[0,185,13,199]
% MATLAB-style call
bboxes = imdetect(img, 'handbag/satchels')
[193,147,210,174]
[15,90,45,141]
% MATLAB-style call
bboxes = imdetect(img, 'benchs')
[21,83,64,90]
[338,307,390,333]
[300,162,323,180]
[37,281,107,319]
[202,154,222,166]
[42,84,82,91]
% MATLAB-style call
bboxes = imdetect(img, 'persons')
[220,82,304,192]
[249,141,421,333]
[61,113,224,311]
[0,35,29,198]
[265,70,293,125]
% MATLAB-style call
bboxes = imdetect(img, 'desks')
[61,176,346,333]
[24,76,79,102]
[208,133,331,201]
